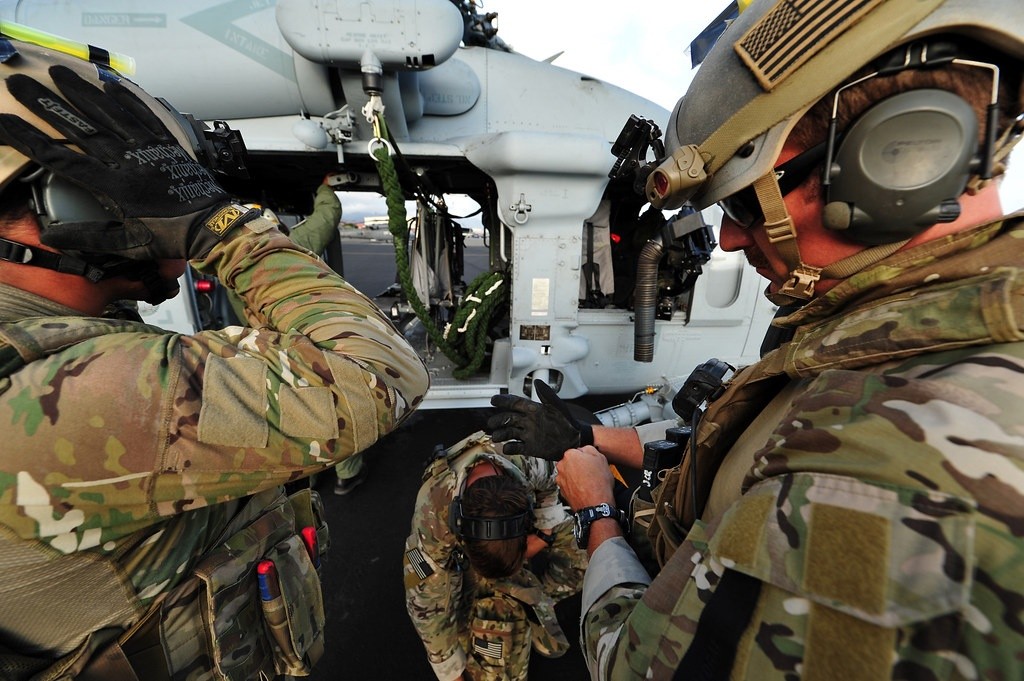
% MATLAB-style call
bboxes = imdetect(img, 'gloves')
[486,378,595,461]
[0,65,232,260]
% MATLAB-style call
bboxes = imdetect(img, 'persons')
[229,173,343,328]
[484,0,1024,681]
[0,33,432,681]
[403,429,588,681]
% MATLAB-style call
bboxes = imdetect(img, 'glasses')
[717,131,847,226]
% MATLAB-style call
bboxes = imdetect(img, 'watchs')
[572,503,630,550]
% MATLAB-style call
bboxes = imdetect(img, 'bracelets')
[532,527,557,550]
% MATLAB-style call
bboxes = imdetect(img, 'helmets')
[0,21,217,306]
[644,0,1024,211]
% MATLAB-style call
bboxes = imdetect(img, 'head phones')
[24,170,128,272]
[820,39,1002,247]
[448,495,536,539]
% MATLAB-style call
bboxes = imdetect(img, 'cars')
[371,281,419,320]
